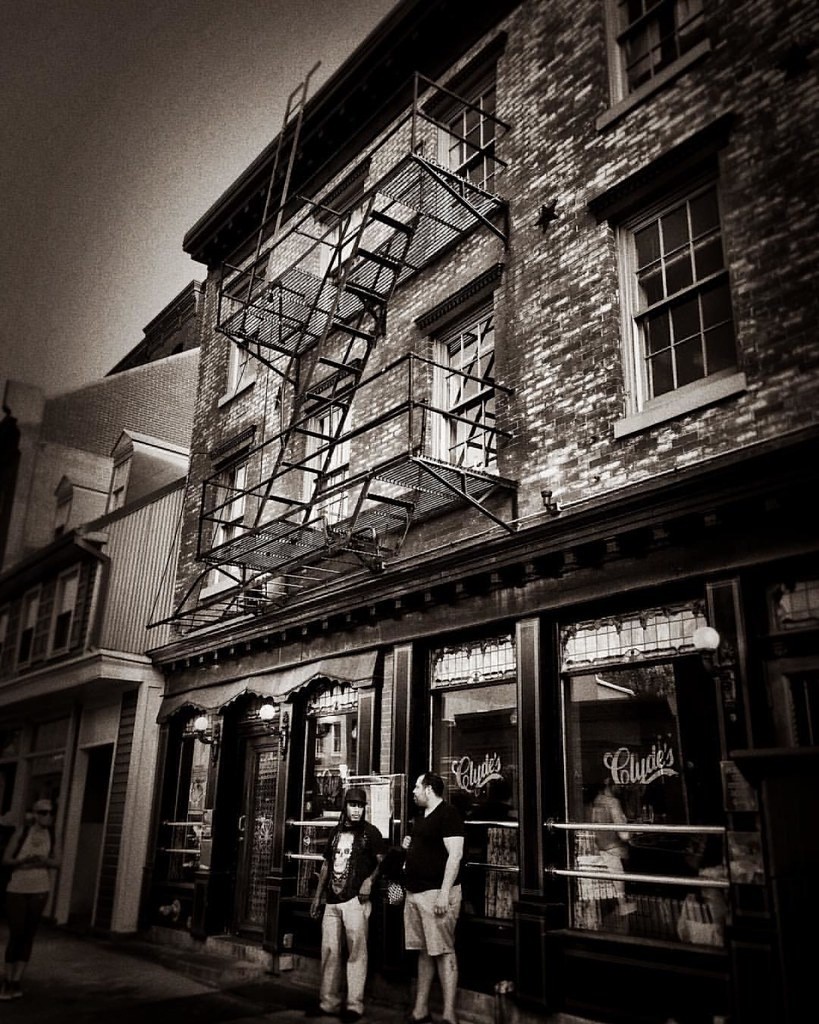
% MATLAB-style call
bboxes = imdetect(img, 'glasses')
[35,810,56,816]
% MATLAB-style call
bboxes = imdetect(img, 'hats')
[346,789,367,804]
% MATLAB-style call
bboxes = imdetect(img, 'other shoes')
[340,1010,364,1024]
[11,980,23,998]
[305,1006,340,1018]
[402,1010,433,1024]
[0,978,12,1000]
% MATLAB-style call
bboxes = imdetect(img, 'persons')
[586,758,637,932]
[309,788,384,1024]
[394,772,469,1024]
[1,798,62,1000]
